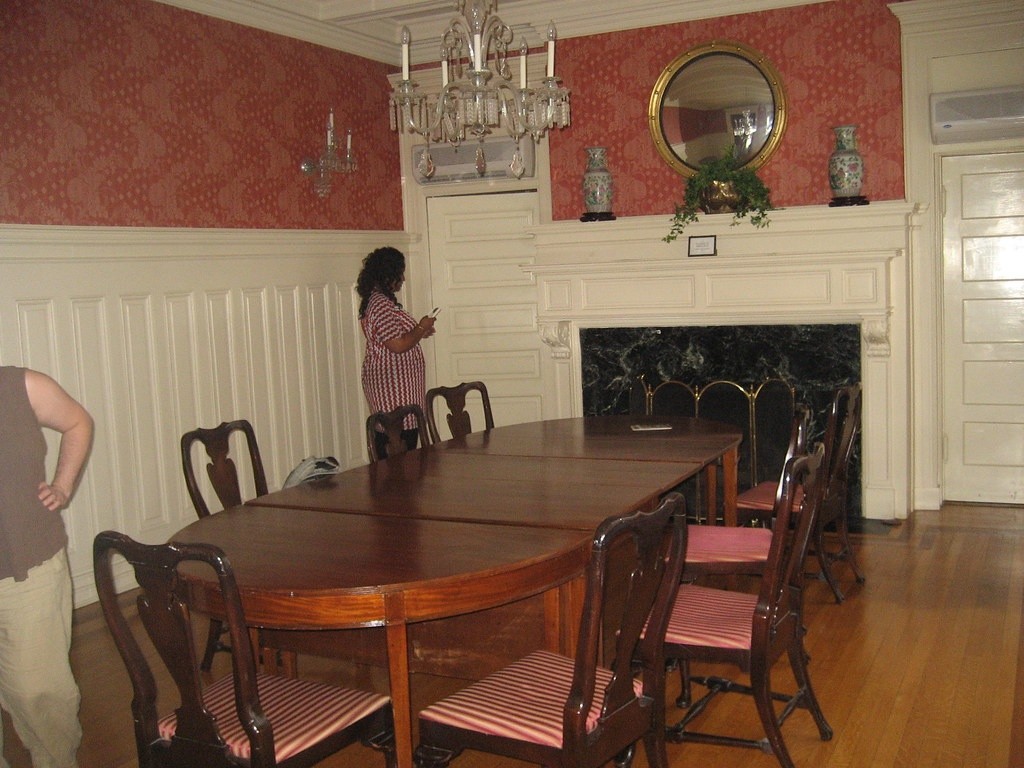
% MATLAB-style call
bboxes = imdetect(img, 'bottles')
[829,125,864,198]
[584,148,613,213]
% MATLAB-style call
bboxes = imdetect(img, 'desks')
[166,413,743,768]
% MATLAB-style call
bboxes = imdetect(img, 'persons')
[0,365,94,768]
[357,248,436,459]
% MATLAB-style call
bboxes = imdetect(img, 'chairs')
[366,404,430,464]
[94,531,397,768]
[180,420,284,674]
[722,382,865,604]
[616,442,832,768]
[413,492,688,768]
[426,380,495,444]
[667,402,813,665]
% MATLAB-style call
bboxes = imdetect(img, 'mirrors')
[647,40,789,180]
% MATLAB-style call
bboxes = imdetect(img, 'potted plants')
[660,143,785,244]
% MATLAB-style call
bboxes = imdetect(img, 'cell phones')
[430,309,440,319]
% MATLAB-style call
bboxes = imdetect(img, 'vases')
[828,126,864,197]
[581,147,613,212]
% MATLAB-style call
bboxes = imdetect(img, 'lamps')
[387,0,571,180]
[299,106,361,203]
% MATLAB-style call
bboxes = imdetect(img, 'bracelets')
[417,325,425,330]
